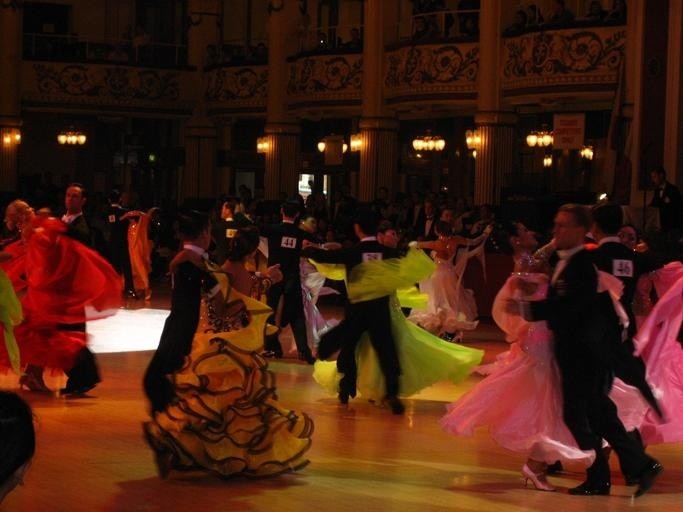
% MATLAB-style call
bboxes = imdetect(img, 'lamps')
[317,142,325,152]
[465,128,476,152]
[411,135,446,151]
[579,145,594,160]
[526,131,554,147]
[257,138,270,153]
[58,131,86,145]
[350,134,360,152]
[14,131,21,144]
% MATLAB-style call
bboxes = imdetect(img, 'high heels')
[451,332,464,343]
[18,372,53,391]
[522,464,556,491]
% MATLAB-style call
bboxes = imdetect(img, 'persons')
[0,163,683,499]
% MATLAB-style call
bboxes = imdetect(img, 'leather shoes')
[567,480,610,495]
[634,463,664,498]
[60,378,102,393]
[262,350,283,358]
